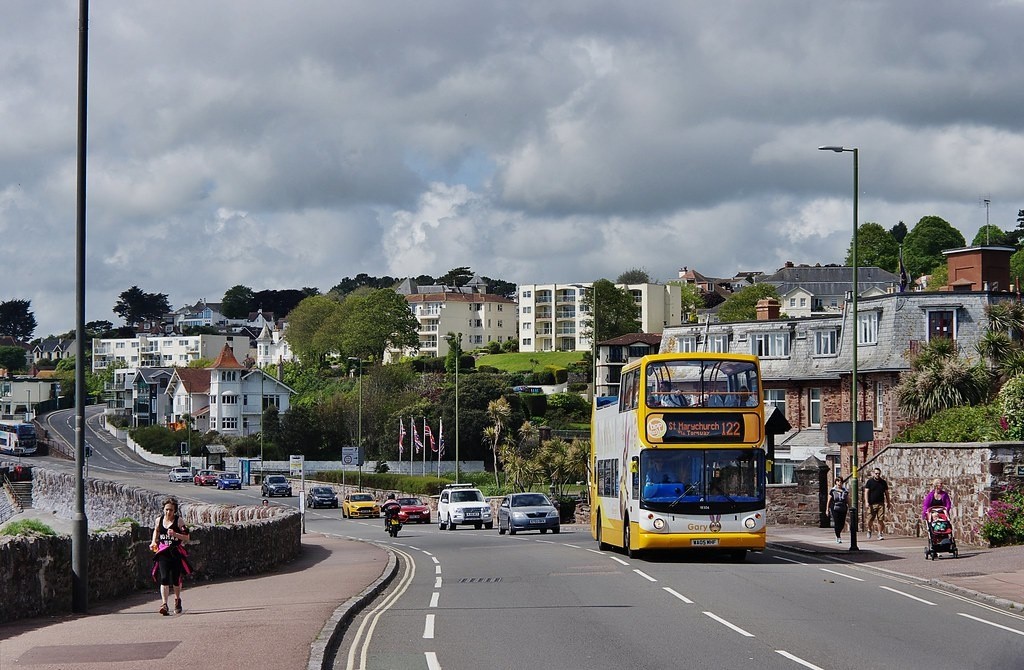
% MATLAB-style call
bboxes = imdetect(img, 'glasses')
[836,480,843,482]
[875,472,881,475]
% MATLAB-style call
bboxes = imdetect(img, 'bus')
[589,352,774,560]
[0,419,39,455]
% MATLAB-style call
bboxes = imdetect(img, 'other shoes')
[384,528,389,531]
[866,529,872,538]
[836,537,842,543]
[875,534,885,541]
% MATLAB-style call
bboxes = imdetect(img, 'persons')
[706,381,757,408]
[262,499,268,506]
[656,380,689,408]
[863,467,893,540]
[921,479,951,558]
[931,513,946,532]
[644,457,678,485]
[826,474,850,544]
[379,490,402,532]
[149,497,190,616]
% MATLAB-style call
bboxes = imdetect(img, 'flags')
[398,421,445,458]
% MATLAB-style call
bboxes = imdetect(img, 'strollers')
[923,505,959,560]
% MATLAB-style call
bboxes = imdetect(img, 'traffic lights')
[85,446,92,457]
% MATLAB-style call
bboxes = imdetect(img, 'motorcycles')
[378,504,402,538]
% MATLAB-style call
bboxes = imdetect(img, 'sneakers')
[159,604,169,616]
[174,598,182,615]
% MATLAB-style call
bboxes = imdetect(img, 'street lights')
[178,379,193,470]
[347,357,362,492]
[439,334,460,484]
[566,283,599,396]
[24,389,30,414]
[38,381,42,413]
[818,143,860,550]
[252,365,263,481]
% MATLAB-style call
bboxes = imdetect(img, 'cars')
[306,486,339,508]
[497,492,562,536]
[168,468,194,483]
[396,497,431,523]
[341,493,382,519]
[193,468,243,490]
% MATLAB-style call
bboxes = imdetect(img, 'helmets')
[388,493,395,499]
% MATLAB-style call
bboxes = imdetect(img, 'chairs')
[707,394,724,407]
[724,394,741,407]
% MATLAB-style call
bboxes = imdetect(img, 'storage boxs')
[387,505,400,513]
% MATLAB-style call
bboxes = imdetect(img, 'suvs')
[260,475,293,497]
[436,482,494,530]
